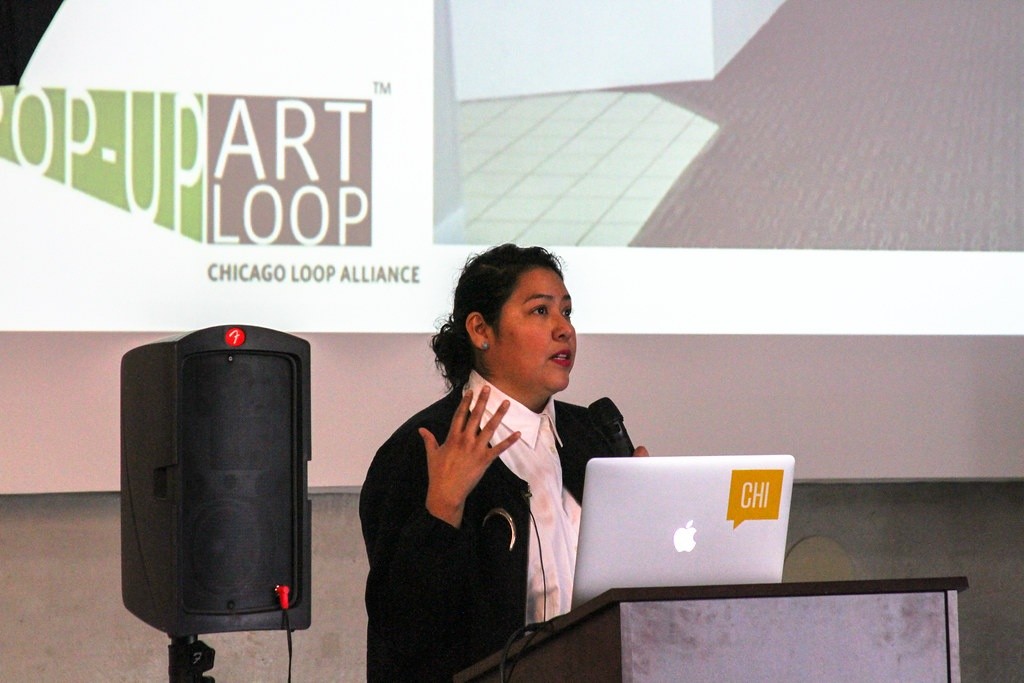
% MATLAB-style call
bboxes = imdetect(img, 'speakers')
[121,323,313,634]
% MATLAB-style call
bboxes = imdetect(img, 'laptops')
[571,455,796,611]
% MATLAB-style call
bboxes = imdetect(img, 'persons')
[359,245,648,682]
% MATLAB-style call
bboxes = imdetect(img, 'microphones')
[587,396,636,457]
